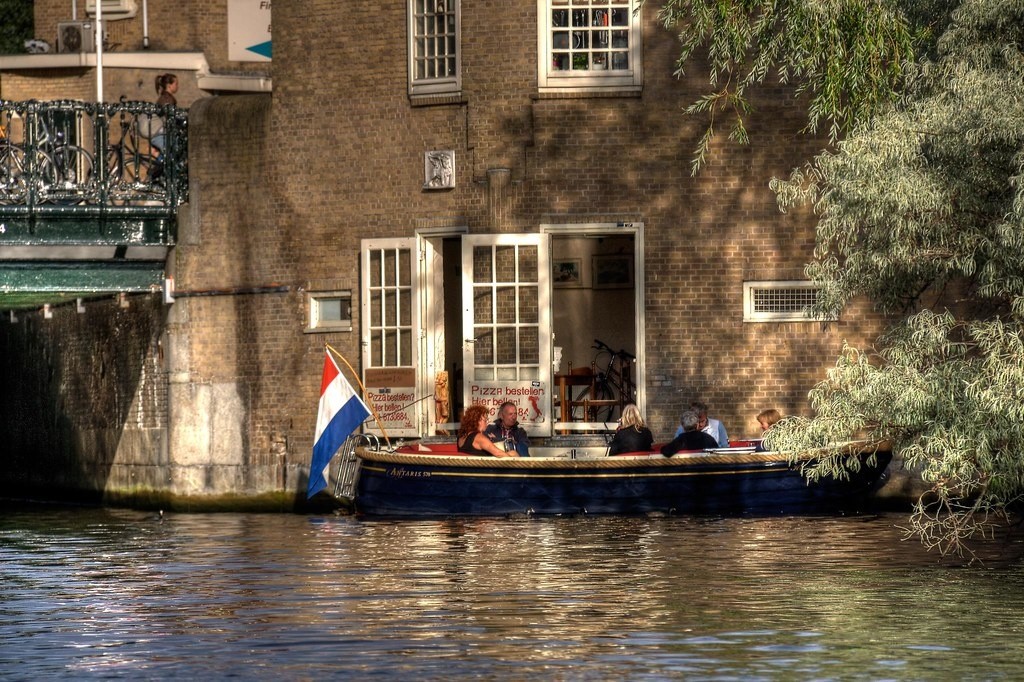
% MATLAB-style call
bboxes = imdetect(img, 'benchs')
[618,439,763,456]
[395,444,475,456]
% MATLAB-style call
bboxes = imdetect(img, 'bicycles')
[0,92,173,208]
[570,338,636,435]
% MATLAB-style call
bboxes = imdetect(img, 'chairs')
[554,360,596,434]
[584,361,631,434]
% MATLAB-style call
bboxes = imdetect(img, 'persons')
[456,403,510,458]
[482,401,531,457]
[143,73,180,184]
[661,401,731,458]
[755,410,783,452]
[608,403,653,456]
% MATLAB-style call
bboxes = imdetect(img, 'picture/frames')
[553,257,584,286]
[591,252,635,291]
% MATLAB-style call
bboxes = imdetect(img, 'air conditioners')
[56,21,94,53]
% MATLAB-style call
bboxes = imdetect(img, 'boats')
[334,423,894,522]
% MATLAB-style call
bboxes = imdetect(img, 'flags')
[305,347,372,500]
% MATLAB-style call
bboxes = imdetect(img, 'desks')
[554,374,597,435]
